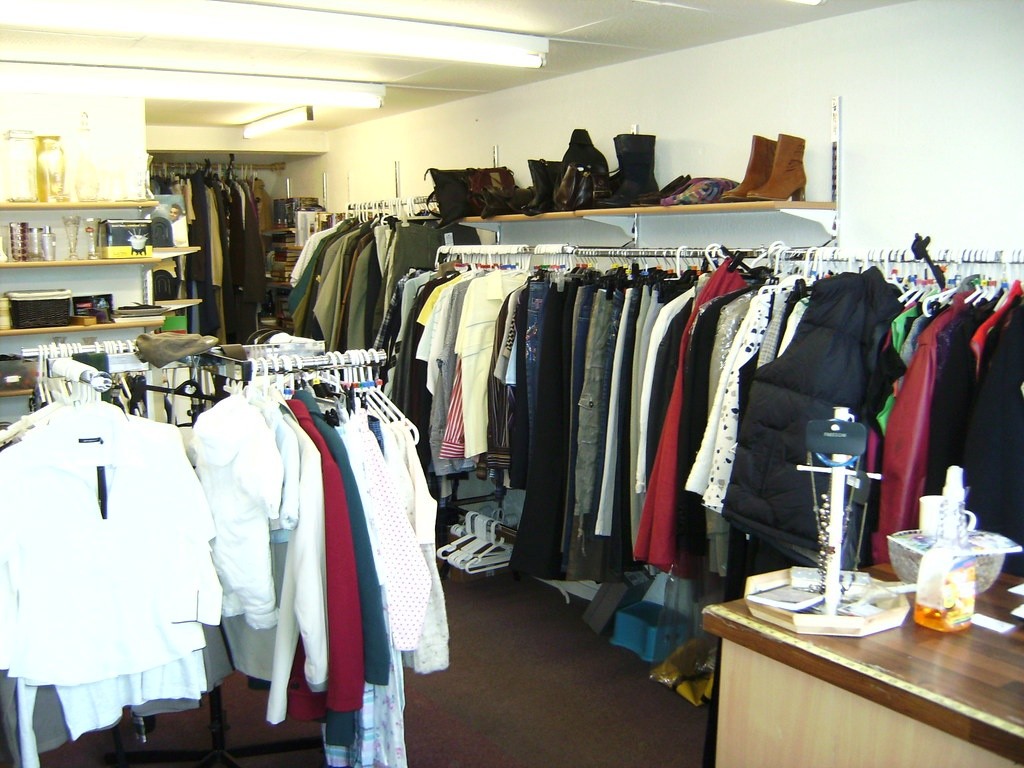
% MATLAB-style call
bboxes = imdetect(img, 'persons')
[169,204,188,247]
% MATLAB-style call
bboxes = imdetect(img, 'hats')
[135,332,219,369]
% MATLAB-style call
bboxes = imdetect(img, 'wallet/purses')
[804,418,868,455]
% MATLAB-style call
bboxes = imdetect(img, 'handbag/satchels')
[422,158,623,232]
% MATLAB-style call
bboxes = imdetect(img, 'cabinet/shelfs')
[0,198,166,397]
[259,229,306,325]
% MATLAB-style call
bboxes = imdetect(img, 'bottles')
[914,465,976,633]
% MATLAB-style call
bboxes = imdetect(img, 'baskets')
[5,287,70,330]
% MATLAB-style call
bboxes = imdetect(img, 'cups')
[10,222,29,261]
[63,215,81,260]
[85,218,100,259]
[29,228,44,261]
[919,495,976,539]
[41,233,56,261]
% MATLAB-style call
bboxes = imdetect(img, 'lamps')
[0,0,550,69]
[147,69,387,110]
[243,106,314,139]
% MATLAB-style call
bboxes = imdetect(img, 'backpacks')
[562,127,610,177]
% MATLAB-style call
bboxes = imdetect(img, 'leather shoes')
[628,173,692,207]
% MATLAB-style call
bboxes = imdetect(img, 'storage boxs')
[608,600,689,664]
[5,289,72,329]
[582,562,655,635]
[95,218,153,260]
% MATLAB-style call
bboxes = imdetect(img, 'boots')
[716,132,807,201]
[595,132,660,207]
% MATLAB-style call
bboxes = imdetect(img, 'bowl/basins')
[888,529,1007,597]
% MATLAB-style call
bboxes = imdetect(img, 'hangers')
[150,160,261,202]
[401,242,1024,318]
[20,330,420,454]
[436,507,514,574]
[341,196,446,229]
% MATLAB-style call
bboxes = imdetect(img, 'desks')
[702,557,1024,768]
